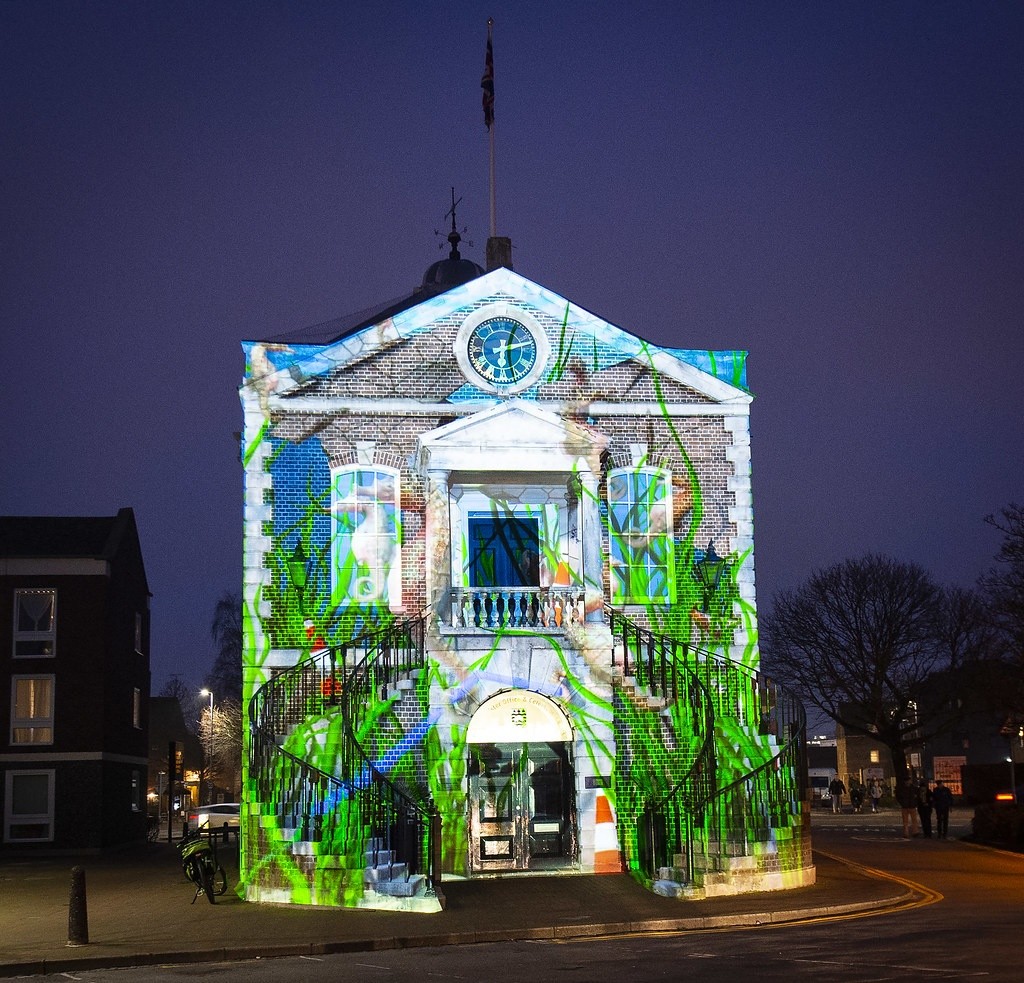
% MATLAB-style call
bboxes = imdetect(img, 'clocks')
[450,300,554,399]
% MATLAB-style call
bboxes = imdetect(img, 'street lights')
[159,771,166,819]
[201,689,213,806]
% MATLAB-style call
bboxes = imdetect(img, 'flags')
[481,26,495,133]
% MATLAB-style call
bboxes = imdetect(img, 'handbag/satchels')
[181,838,217,883]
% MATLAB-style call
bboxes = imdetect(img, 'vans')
[807,768,837,806]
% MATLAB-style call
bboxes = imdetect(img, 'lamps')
[694,536,727,615]
[285,536,316,618]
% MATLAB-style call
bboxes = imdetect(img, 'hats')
[935,779,943,784]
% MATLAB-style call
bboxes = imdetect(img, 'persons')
[930,780,953,841]
[829,773,883,815]
[917,783,934,840]
[896,777,921,838]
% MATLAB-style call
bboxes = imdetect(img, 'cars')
[190,803,240,834]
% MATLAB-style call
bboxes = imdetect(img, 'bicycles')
[175,819,228,905]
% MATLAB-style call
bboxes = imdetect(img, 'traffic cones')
[592,788,628,874]
